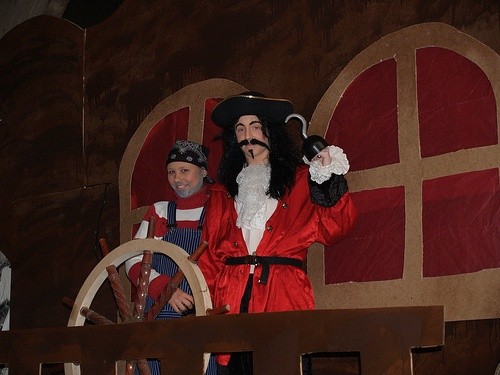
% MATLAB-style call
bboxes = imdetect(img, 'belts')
[225,255,307,285]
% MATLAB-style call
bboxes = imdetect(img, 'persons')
[124,140,217,375]
[197,91,359,375]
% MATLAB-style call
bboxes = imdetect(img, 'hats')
[211,90,295,127]
[165,140,216,185]
